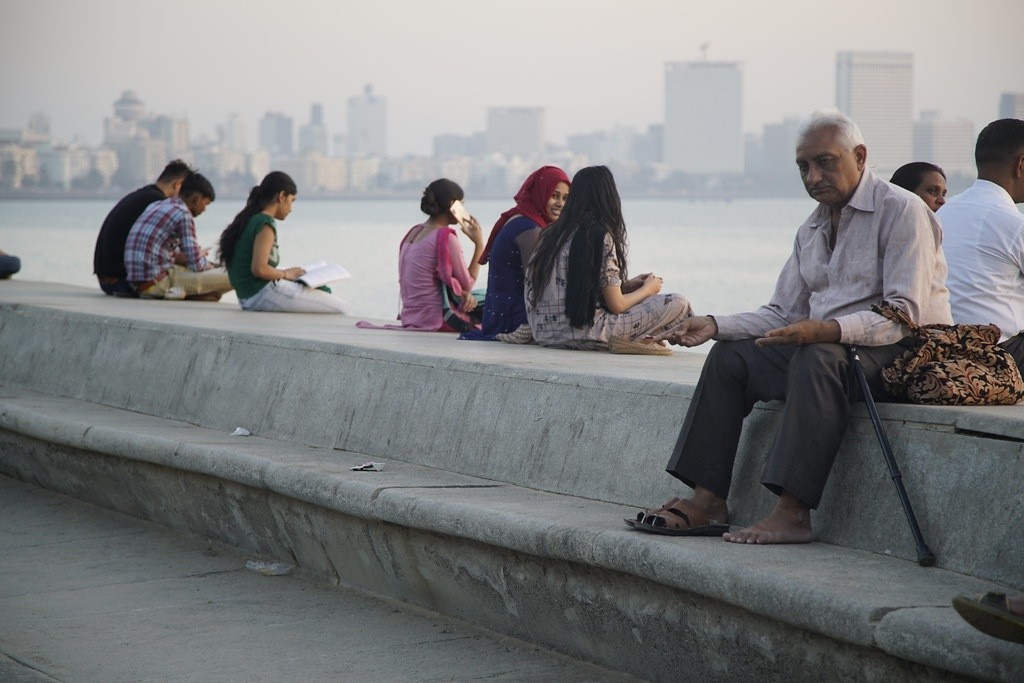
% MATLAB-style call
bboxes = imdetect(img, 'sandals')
[952,591,1024,645]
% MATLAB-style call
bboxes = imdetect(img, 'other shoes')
[607,335,673,355]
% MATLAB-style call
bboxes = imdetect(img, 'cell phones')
[450,200,474,229]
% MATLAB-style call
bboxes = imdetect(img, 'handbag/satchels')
[442,276,486,333]
[871,299,1024,405]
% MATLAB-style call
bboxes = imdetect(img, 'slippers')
[624,497,680,526]
[634,498,730,536]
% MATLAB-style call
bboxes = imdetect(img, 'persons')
[354,178,489,332]
[0,249,20,280]
[123,172,236,300]
[456,166,571,342]
[652,109,953,544]
[524,165,695,352]
[215,171,348,313]
[889,162,947,213]
[923,118,1024,384]
[93,159,209,298]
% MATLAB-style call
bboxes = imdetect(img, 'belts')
[135,271,168,292]
